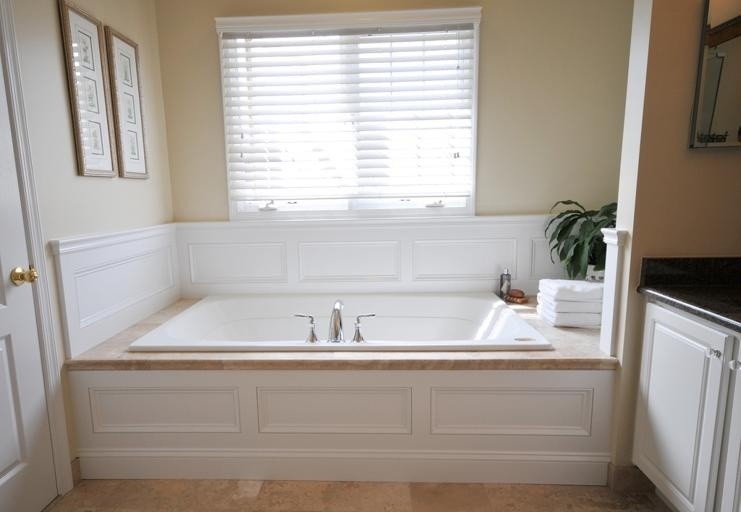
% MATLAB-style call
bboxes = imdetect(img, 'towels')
[537,279,603,328]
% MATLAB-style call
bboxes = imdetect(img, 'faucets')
[327,299,343,344]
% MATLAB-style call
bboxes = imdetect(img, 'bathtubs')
[127,293,553,352]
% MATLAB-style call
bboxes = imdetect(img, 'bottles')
[500,266,512,300]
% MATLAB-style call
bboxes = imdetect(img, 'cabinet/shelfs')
[632,301,741,512]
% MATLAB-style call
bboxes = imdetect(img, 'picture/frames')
[104,26,149,178]
[58,0,119,178]
